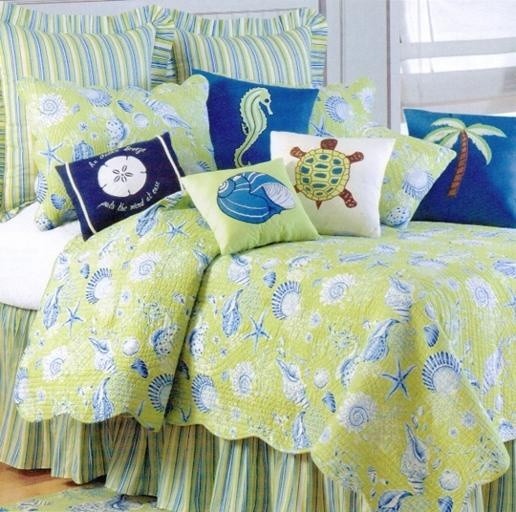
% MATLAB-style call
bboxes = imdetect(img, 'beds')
[0,202,516,512]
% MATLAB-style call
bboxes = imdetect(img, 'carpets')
[0,482,172,512]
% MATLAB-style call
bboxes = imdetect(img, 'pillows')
[400,105,514,230]
[345,77,376,122]
[14,73,219,230]
[179,156,323,253]
[309,83,457,231]
[1,1,176,222]
[54,131,186,243]
[269,130,396,240]
[190,68,320,170]
[163,6,329,91]
